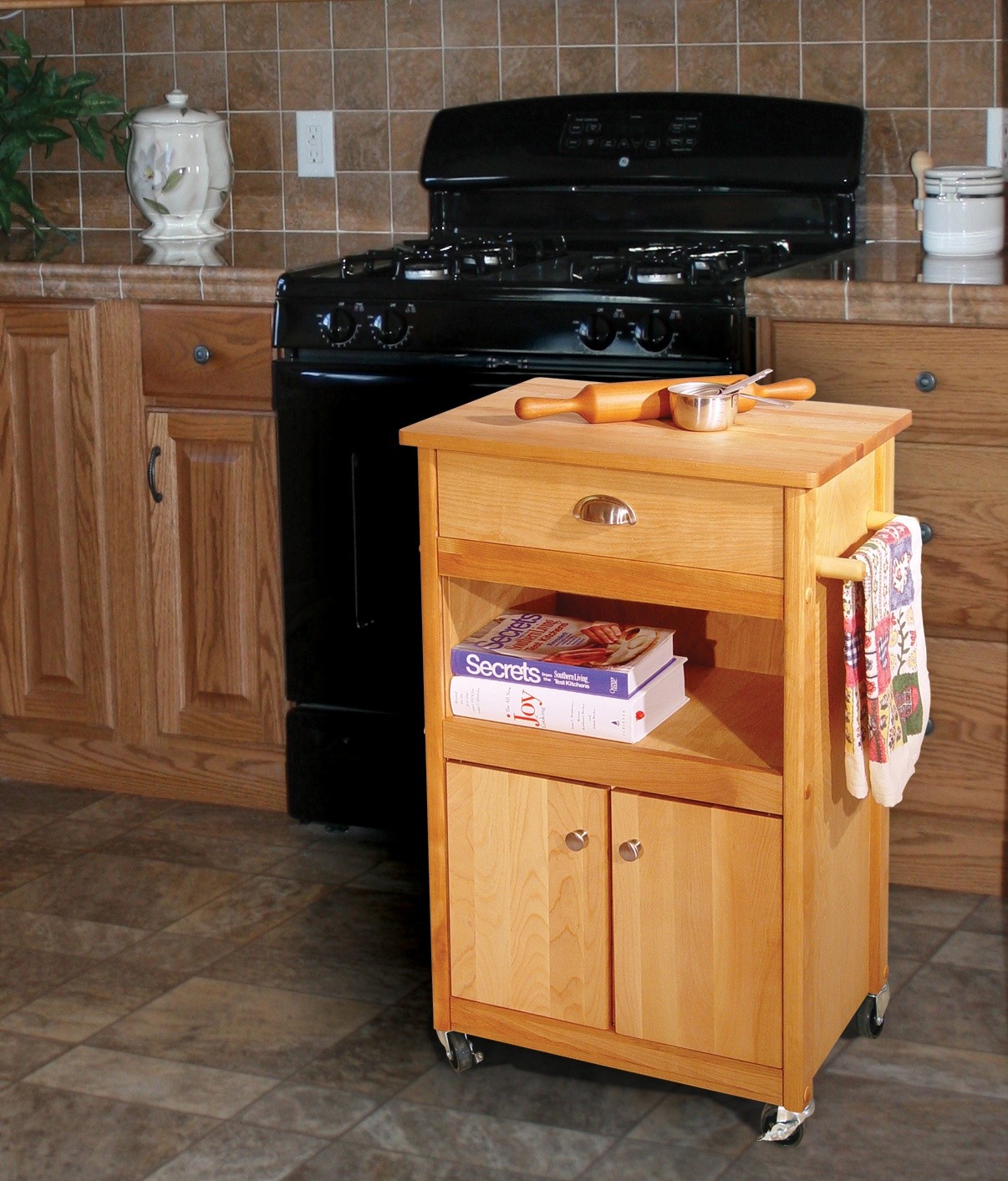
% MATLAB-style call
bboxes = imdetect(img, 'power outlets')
[296,111,335,177]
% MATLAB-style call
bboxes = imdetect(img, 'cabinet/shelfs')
[757,315,1008,896]
[0,302,286,814]
[401,377,913,1147]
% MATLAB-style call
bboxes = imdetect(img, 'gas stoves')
[273,91,868,377]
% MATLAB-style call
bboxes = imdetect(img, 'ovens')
[271,358,675,835]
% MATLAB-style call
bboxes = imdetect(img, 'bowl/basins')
[668,382,741,432]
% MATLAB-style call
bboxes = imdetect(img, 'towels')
[838,515,933,811]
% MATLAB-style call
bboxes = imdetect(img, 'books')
[450,608,691,743]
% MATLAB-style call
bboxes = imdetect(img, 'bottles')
[125,86,236,241]
[913,165,1006,259]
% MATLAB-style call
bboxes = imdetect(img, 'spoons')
[911,150,933,231]
